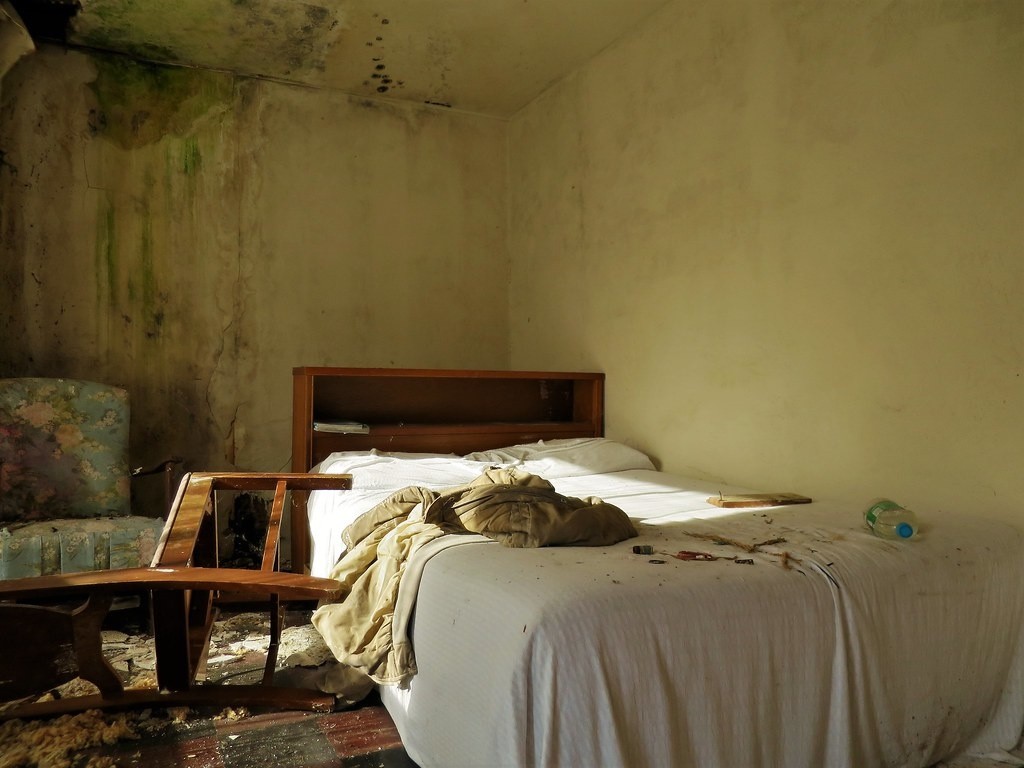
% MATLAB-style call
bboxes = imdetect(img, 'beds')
[291,367,1024,768]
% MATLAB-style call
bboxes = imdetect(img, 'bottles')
[863,496,918,539]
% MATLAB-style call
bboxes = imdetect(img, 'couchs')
[0,377,167,636]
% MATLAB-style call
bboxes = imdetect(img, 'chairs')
[0,472,353,728]
